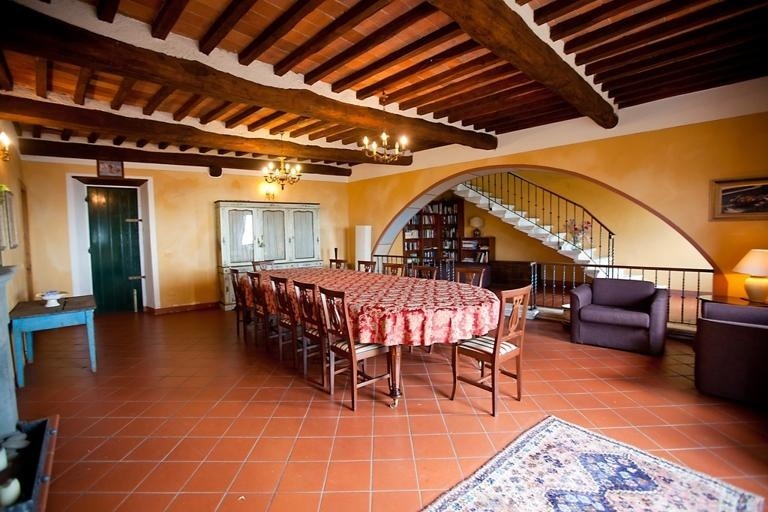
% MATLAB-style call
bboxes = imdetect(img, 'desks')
[696,294,767,318]
[9,294,99,389]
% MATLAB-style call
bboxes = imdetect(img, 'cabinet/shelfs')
[459,236,496,287]
[214,200,324,312]
[402,196,465,281]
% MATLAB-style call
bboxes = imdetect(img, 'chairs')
[292,280,368,389]
[229,269,255,344]
[381,262,407,277]
[269,275,311,369]
[318,285,392,412]
[357,260,376,273]
[454,266,485,288]
[329,258,348,269]
[412,264,437,280]
[449,284,533,417]
[245,271,286,354]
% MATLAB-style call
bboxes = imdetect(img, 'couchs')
[694,300,768,405]
[362,90,410,165]
[568,277,669,356]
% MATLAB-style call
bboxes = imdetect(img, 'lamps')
[469,215,484,236]
[0,131,13,162]
[731,248,768,306]
[261,155,304,191]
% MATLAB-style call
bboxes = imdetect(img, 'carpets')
[415,410,765,511]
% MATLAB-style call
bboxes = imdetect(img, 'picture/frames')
[96,158,126,180]
[707,175,768,222]
[4,189,19,249]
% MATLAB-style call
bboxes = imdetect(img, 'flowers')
[563,216,593,250]
